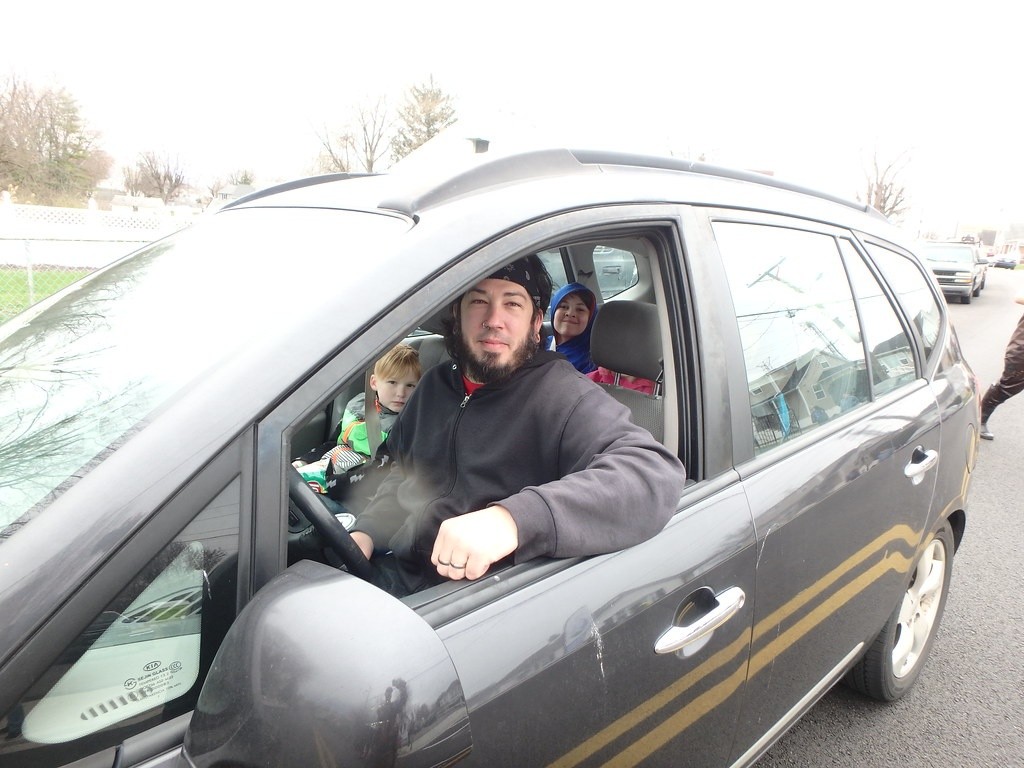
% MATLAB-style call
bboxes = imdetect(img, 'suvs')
[0,131,986,768]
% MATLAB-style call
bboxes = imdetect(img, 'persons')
[585,366,662,395]
[980,295,1024,440]
[348,254,686,600]
[542,281,599,374]
[292,344,421,493]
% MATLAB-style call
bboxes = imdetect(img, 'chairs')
[324,304,454,441]
[591,300,665,445]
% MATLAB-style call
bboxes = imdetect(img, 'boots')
[981,384,1010,440]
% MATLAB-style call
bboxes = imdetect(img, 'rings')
[438,556,450,566]
[450,561,466,569]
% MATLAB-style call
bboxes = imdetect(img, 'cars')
[989,255,1016,270]
[923,245,986,306]
